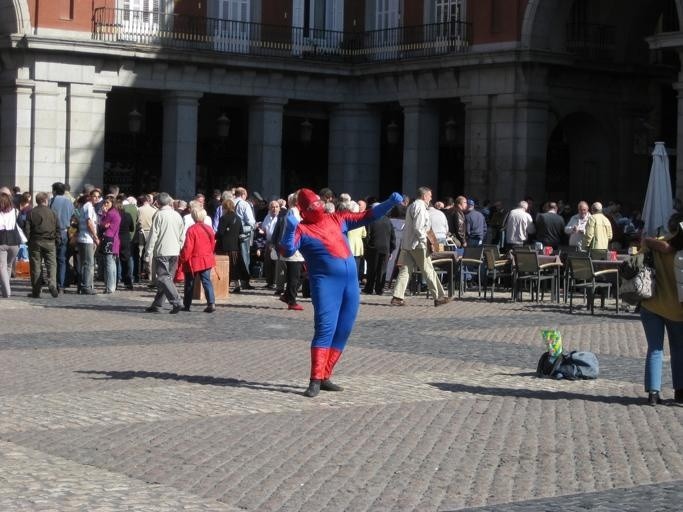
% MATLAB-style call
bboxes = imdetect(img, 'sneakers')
[435,297,452,307]
[231,283,311,310]
[321,380,344,391]
[391,297,405,306]
[306,379,321,398]
[1,274,216,314]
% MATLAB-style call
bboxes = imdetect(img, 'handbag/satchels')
[98,236,112,255]
[617,262,658,305]
[537,351,600,381]
[15,222,28,244]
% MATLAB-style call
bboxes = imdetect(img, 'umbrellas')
[640,141,671,229]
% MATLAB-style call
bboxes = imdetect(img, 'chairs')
[408,243,634,313]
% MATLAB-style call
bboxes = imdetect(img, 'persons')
[1,182,312,313]
[640,215,682,405]
[266,187,401,398]
[319,187,683,307]
